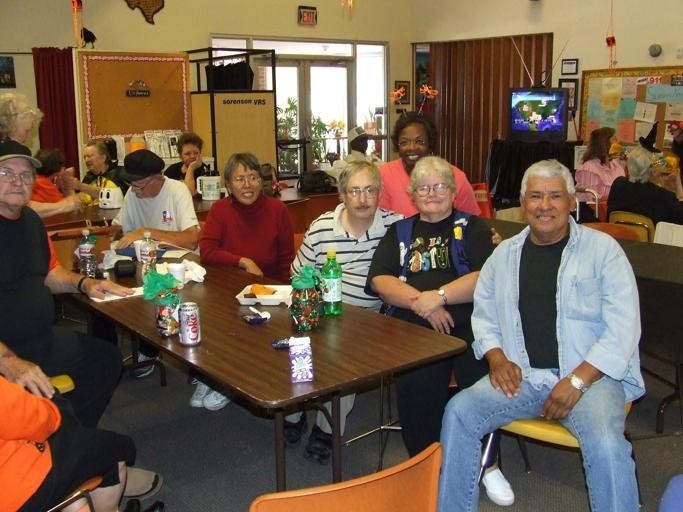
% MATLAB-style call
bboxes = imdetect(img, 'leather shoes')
[283,410,308,449]
[122,498,140,512]
[302,424,333,466]
[141,500,165,512]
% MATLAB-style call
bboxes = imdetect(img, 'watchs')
[566,371,590,395]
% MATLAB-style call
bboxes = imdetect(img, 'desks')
[0,187,338,250]
[486,139,583,204]
[47,233,465,496]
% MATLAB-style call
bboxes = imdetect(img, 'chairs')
[45,472,102,512]
[482,187,682,438]
[376,300,501,476]
[252,440,443,510]
[478,398,632,511]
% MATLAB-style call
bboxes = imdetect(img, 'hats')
[639,119,663,154]
[348,126,367,145]
[0,139,43,168]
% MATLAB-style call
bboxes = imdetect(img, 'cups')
[166,262,187,290]
[195,176,221,201]
[113,260,136,278]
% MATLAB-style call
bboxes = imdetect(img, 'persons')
[607,140,683,227]
[106,149,198,379]
[576,128,628,205]
[288,114,521,505]
[26,137,129,217]
[439,159,653,512]
[163,132,210,199]
[658,474,682,512]
[0,137,165,512]
[189,151,292,411]
[668,121,683,165]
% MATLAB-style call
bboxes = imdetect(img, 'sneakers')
[122,465,164,500]
[202,389,233,412]
[480,466,515,506]
[134,352,163,377]
[188,381,213,409]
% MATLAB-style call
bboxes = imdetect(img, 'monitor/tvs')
[508,88,569,142]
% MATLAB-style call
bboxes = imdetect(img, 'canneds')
[179,302,201,346]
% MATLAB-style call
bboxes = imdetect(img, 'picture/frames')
[394,80,410,106]
[558,77,580,112]
[561,58,578,75]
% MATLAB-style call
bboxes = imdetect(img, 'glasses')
[396,139,428,148]
[226,174,262,185]
[413,182,450,198]
[343,186,377,198]
[123,178,153,191]
[0,168,36,185]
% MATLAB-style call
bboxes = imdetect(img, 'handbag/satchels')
[296,169,339,195]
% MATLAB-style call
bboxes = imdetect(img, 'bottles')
[138,230,156,280]
[319,249,343,316]
[78,229,98,279]
[288,276,320,332]
